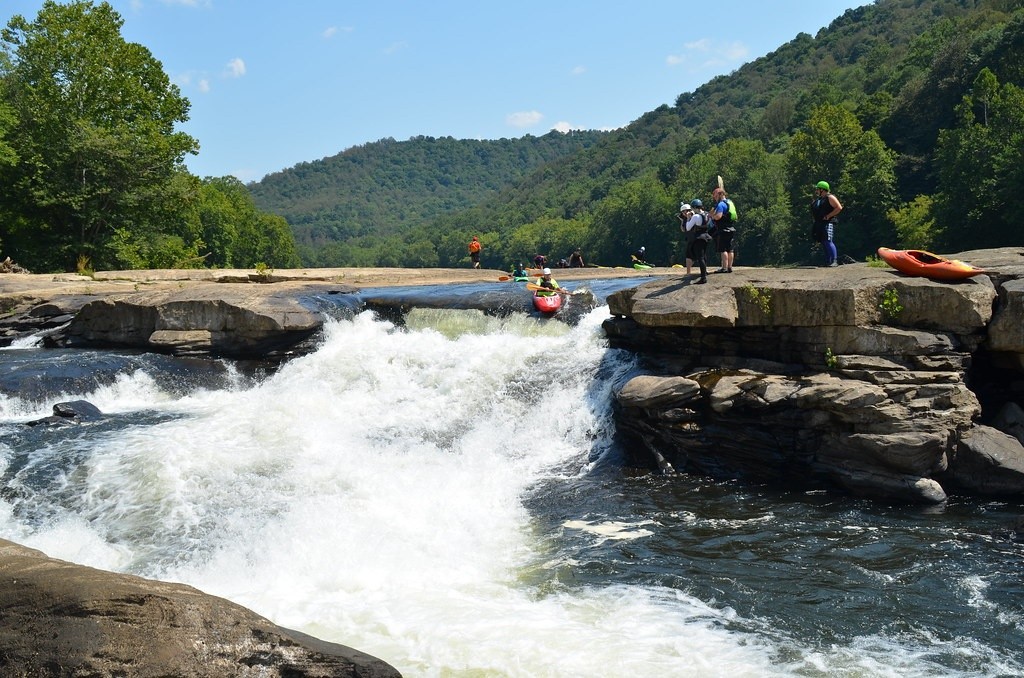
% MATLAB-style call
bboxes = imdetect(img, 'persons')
[678,188,736,284]
[536,268,565,296]
[635,247,647,265]
[532,256,548,269]
[807,181,842,267]
[468,236,481,268]
[554,249,582,268]
[511,263,528,278]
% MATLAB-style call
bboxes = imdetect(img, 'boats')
[634,264,652,269]
[514,276,530,282]
[534,290,561,312]
[878,246,986,278]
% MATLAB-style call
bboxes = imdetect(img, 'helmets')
[814,180,830,191]
[543,268,552,276]
[680,204,692,213]
[518,263,523,267]
[473,237,478,241]
[640,247,646,251]
[712,187,725,198]
[690,198,703,207]
[576,247,581,250]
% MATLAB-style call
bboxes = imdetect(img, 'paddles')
[631,255,655,267]
[588,262,598,266]
[498,276,515,281]
[527,283,570,295]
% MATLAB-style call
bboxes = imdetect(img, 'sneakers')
[714,267,733,273]
[828,259,838,267]
[694,275,707,285]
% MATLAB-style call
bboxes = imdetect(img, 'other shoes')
[472,264,477,269]
